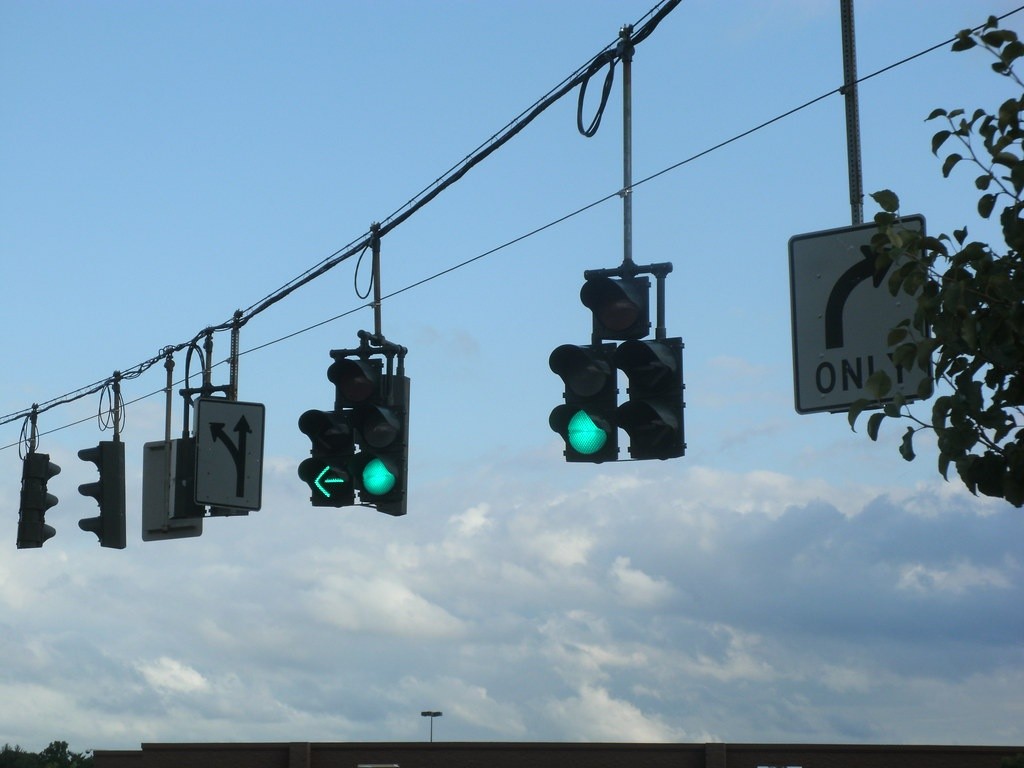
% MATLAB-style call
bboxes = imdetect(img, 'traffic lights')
[321,355,395,408]
[581,263,674,342]
[356,404,414,511]
[298,411,350,511]
[617,338,687,460]
[549,343,618,466]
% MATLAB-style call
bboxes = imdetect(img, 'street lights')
[78,441,126,549]
[16,453,61,549]
[421,711,443,742]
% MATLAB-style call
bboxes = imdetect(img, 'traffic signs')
[788,213,934,415]
